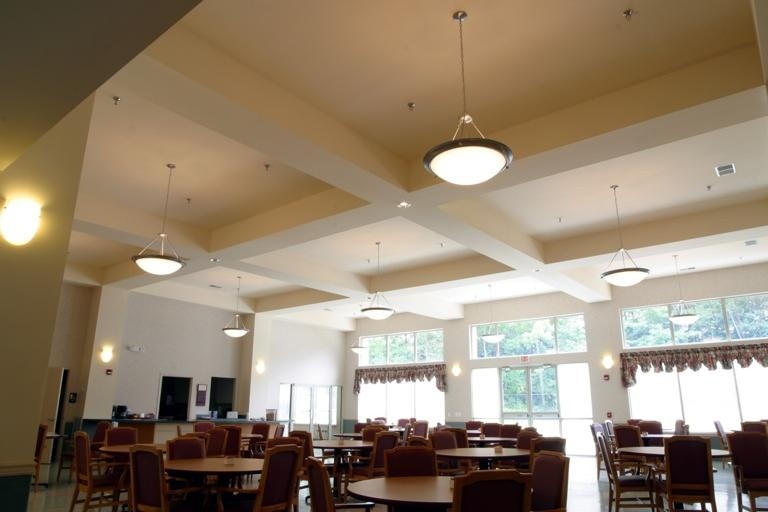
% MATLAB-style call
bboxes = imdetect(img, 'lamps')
[360,237,395,320]
[599,180,652,289]
[420,9,517,186]
[349,303,369,356]
[131,160,186,276]
[480,283,505,344]
[667,253,702,327]
[221,275,250,339]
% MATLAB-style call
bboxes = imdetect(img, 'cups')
[495,446,503,452]
[224,456,235,465]
[112,421,118,428]
[479,434,485,439]
[337,439,344,445]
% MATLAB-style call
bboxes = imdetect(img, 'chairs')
[590,417,768,511]
[29,418,568,510]
